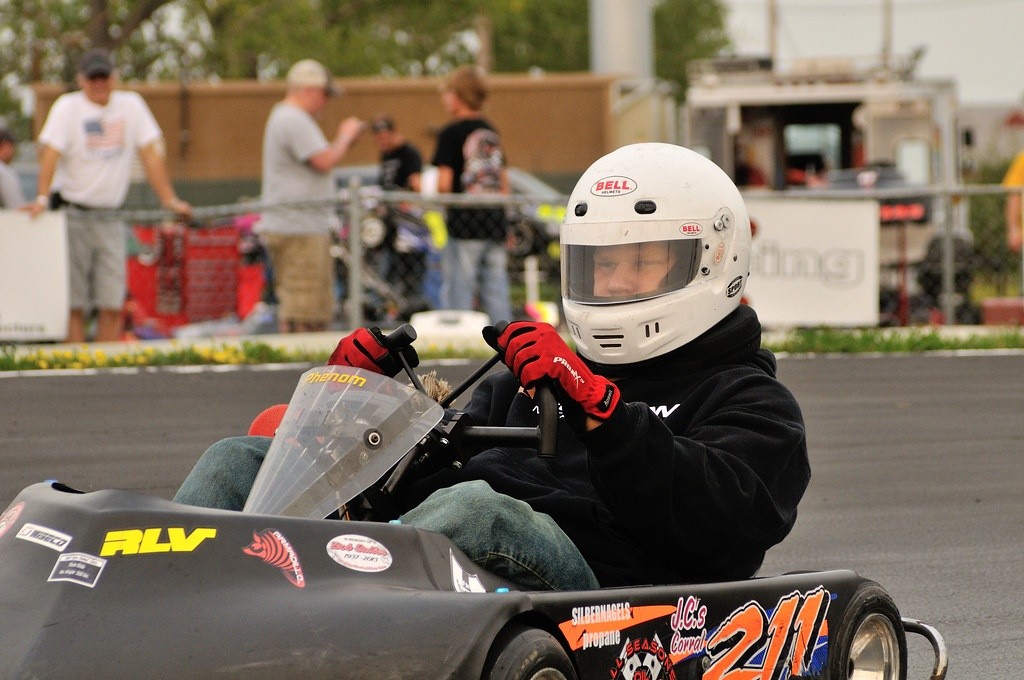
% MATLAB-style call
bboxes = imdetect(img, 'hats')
[446,69,488,112]
[79,51,111,73]
[285,58,345,95]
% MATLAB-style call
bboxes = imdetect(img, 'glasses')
[87,69,110,82]
[323,88,334,99]
[371,123,390,130]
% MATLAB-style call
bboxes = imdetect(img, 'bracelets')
[34,193,50,207]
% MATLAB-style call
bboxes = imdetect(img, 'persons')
[255,59,365,335]
[170,141,812,591]
[0,130,26,209]
[372,119,423,194]
[19,48,198,344]
[432,61,512,324]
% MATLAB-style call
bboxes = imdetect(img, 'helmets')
[561,140,750,364]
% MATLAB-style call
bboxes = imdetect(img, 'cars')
[323,165,568,265]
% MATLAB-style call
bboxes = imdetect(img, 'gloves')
[325,325,419,378]
[481,321,621,422]
